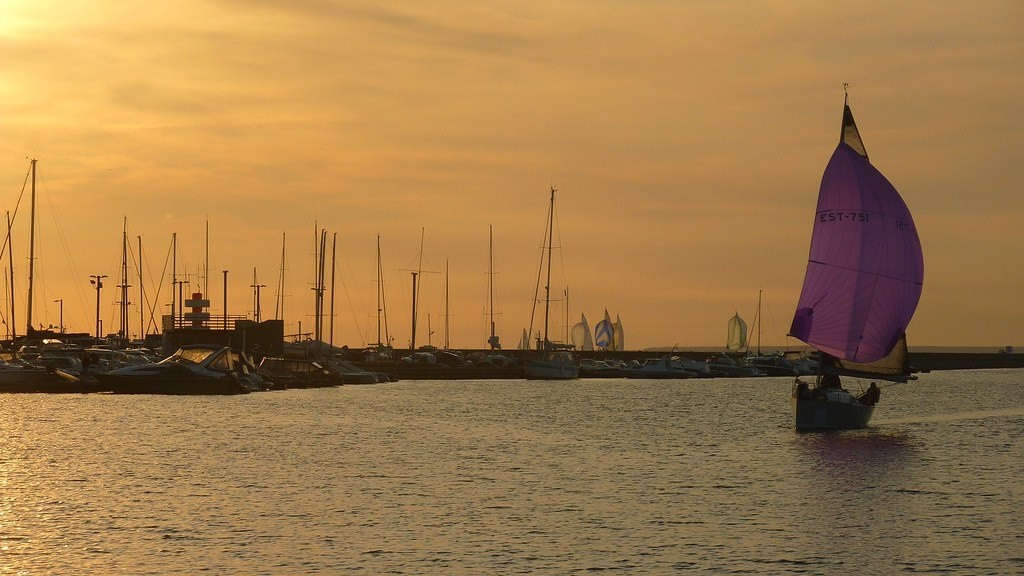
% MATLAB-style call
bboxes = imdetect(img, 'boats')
[579,361,620,378]
[672,291,819,378]
[618,358,689,378]
[94,344,273,395]
[258,356,340,388]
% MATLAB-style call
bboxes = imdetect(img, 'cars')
[400,353,438,365]
[361,350,388,361]
[18,346,40,359]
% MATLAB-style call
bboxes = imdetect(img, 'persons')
[856,382,878,404]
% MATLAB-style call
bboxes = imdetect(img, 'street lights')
[223,271,229,347]
[53,299,62,333]
[172,281,189,328]
[117,285,132,339]
[90,275,108,345]
[411,273,417,365]
[251,285,266,323]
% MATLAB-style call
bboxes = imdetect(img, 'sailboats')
[468,225,518,378]
[0,159,158,384]
[438,259,468,366]
[524,189,582,378]
[784,92,931,431]
